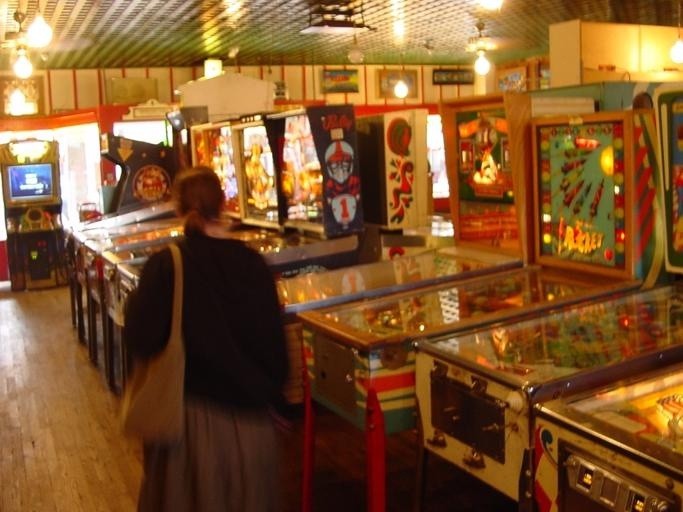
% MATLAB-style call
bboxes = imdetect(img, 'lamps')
[4,0,53,79]
[670,0,683,64]
[464,21,498,75]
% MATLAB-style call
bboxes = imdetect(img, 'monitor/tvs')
[5,162,55,203]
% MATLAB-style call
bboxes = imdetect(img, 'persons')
[120,164,292,510]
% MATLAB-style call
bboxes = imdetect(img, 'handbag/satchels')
[119,342,185,444]
